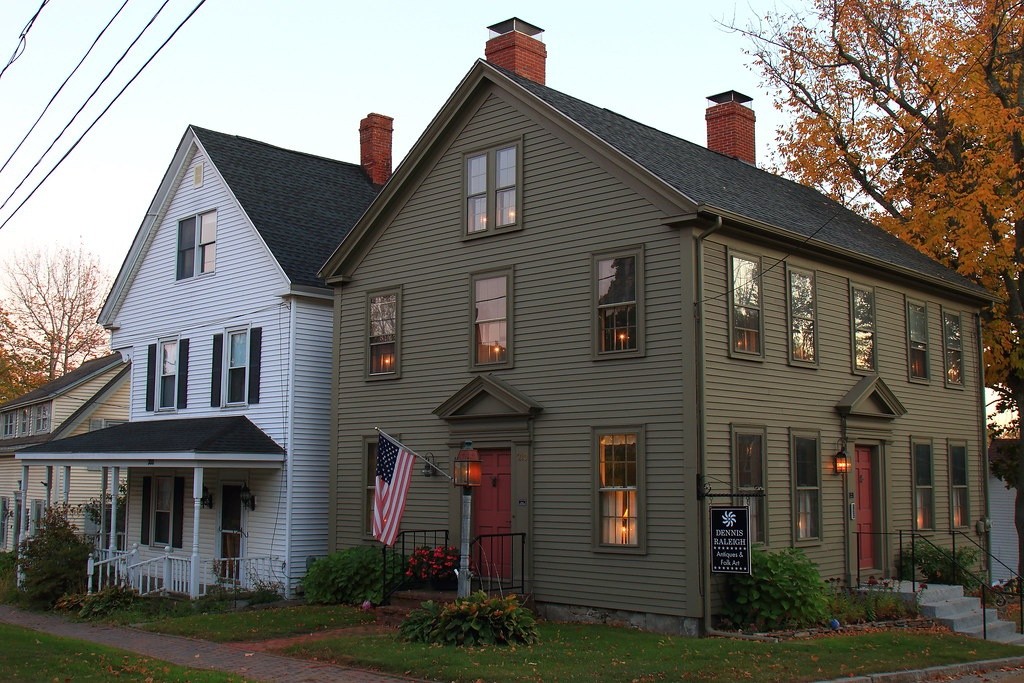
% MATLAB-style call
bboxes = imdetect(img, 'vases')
[436,578,458,591]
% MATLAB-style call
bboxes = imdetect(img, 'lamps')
[833,437,847,473]
[200,483,213,509]
[422,452,440,477]
[240,482,255,510]
[453,440,484,495]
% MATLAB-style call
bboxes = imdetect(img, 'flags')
[371,433,415,548]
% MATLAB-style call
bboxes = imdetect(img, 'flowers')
[405,546,460,584]
